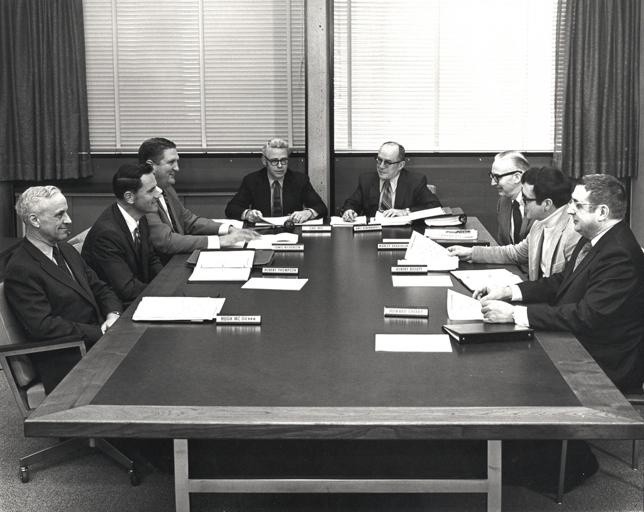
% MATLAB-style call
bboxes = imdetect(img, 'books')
[368,207,467,227]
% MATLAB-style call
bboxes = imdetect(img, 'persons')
[225,138,328,223]
[138,138,260,267]
[339,141,443,222]
[5,185,173,470]
[490,151,530,281]
[472,174,644,493]
[446,166,583,281]
[82,162,164,318]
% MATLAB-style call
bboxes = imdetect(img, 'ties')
[381,180,393,210]
[572,240,592,271]
[272,180,282,217]
[133,228,143,264]
[161,189,180,233]
[510,200,522,243]
[52,246,73,278]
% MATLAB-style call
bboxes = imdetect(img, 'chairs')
[555,392,643,505]
[0,282,141,486]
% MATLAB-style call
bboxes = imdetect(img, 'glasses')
[264,155,287,168]
[374,158,403,168]
[521,187,538,204]
[487,171,522,182]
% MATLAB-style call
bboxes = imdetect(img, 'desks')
[22,216,643,511]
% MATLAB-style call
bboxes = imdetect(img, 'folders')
[188,249,275,269]
[442,323,534,345]
